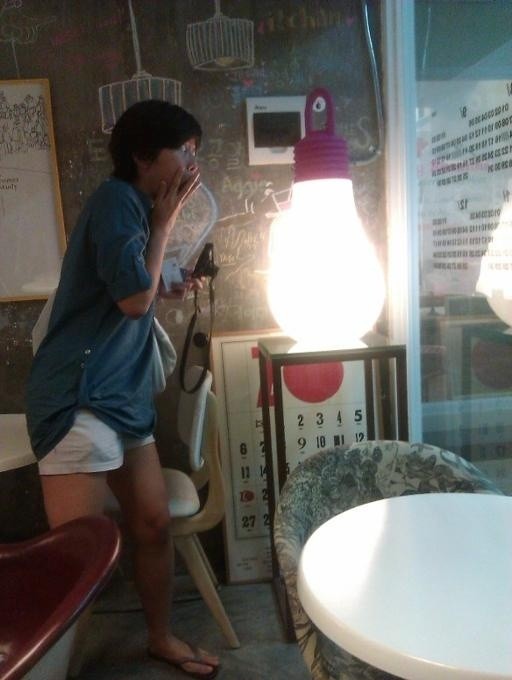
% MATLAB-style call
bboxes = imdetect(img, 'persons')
[23,98,222,680]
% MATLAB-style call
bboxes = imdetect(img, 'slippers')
[147,639,219,679]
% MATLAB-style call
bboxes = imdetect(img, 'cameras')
[194,244,221,277]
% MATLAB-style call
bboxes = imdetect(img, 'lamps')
[96,1,257,142]
[261,84,391,347]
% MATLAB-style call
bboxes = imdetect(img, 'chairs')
[0,502,130,680]
[66,365,241,679]
[267,438,508,679]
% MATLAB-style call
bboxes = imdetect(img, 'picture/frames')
[201,326,387,584]
[0,76,69,305]
